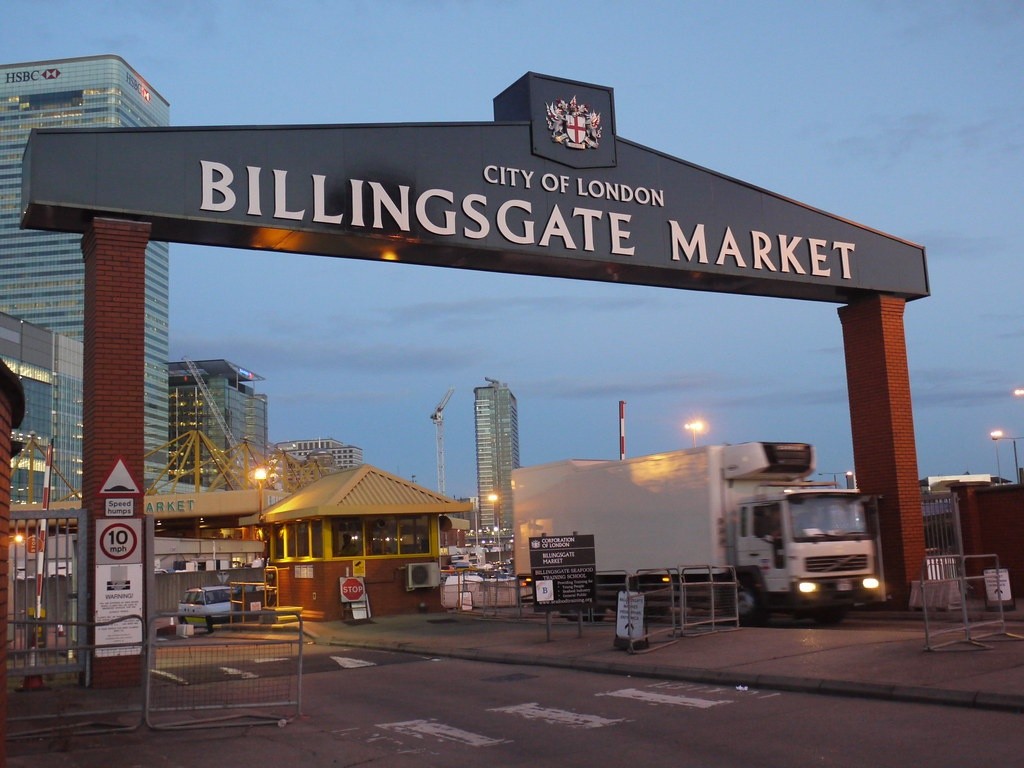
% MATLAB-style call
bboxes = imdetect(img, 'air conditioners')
[405,562,440,588]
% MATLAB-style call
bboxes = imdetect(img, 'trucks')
[511,442,875,627]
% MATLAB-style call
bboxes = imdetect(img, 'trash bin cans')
[236,591,263,621]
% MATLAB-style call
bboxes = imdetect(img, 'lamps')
[254,468,267,523]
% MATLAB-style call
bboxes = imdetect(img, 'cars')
[441,555,481,571]
[178,586,238,617]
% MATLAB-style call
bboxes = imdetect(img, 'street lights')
[991,431,1003,483]
[489,495,501,562]
[691,423,703,448]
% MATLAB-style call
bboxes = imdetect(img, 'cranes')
[184,357,251,472]
[430,388,454,496]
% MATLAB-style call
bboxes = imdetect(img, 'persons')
[756,506,782,538]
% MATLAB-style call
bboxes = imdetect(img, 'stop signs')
[340,577,366,602]
